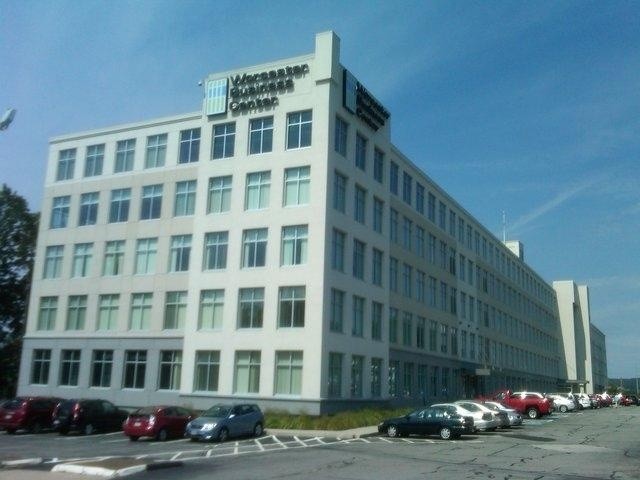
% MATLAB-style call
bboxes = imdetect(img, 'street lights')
[0,108,17,130]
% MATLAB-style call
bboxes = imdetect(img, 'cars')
[122,406,198,443]
[374,389,639,441]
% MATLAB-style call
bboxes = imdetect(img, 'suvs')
[51,398,130,436]
[0,395,63,435]
[185,402,264,443]
